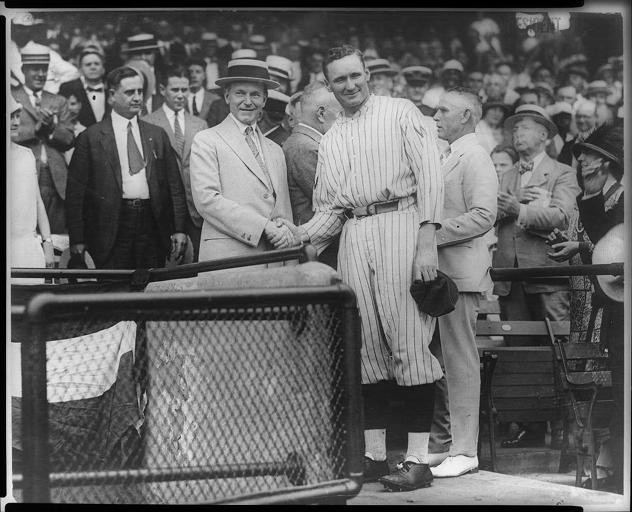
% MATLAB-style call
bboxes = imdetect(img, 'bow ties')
[87,86,103,92]
[519,161,534,175]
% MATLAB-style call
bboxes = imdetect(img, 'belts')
[124,199,151,207]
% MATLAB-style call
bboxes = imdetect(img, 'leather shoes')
[429,454,480,478]
[339,457,389,483]
[420,451,449,465]
[501,429,543,448]
[380,462,433,490]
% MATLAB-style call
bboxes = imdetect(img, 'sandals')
[580,466,621,489]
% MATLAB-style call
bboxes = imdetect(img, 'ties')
[127,121,147,174]
[193,95,200,116]
[32,91,42,111]
[174,111,185,159]
[245,127,273,188]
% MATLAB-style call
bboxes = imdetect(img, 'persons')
[405,83,500,482]
[265,45,443,491]
[189,57,295,276]
[6,5,625,495]
[66,66,190,301]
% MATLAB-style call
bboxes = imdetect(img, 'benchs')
[470,298,624,474]
[541,316,624,491]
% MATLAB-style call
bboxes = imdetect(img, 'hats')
[504,104,559,139]
[534,86,556,103]
[20,45,50,65]
[410,271,459,316]
[121,34,162,53]
[572,118,624,167]
[215,60,281,89]
[58,245,98,285]
[583,80,612,96]
[12,13,35,26]
[126,59,156,102]
[288,91,302,104]
[402,66,431,87]
[265,55,295,82]
[482,96,513,114]
[559,63,590,78]
[232,49,258,59]
[10,92,23,115]
[591,224,624,302]
[365,59,398,76]
[248,34,269,50]
[268,90,292,104]
[443,59,463,73]
